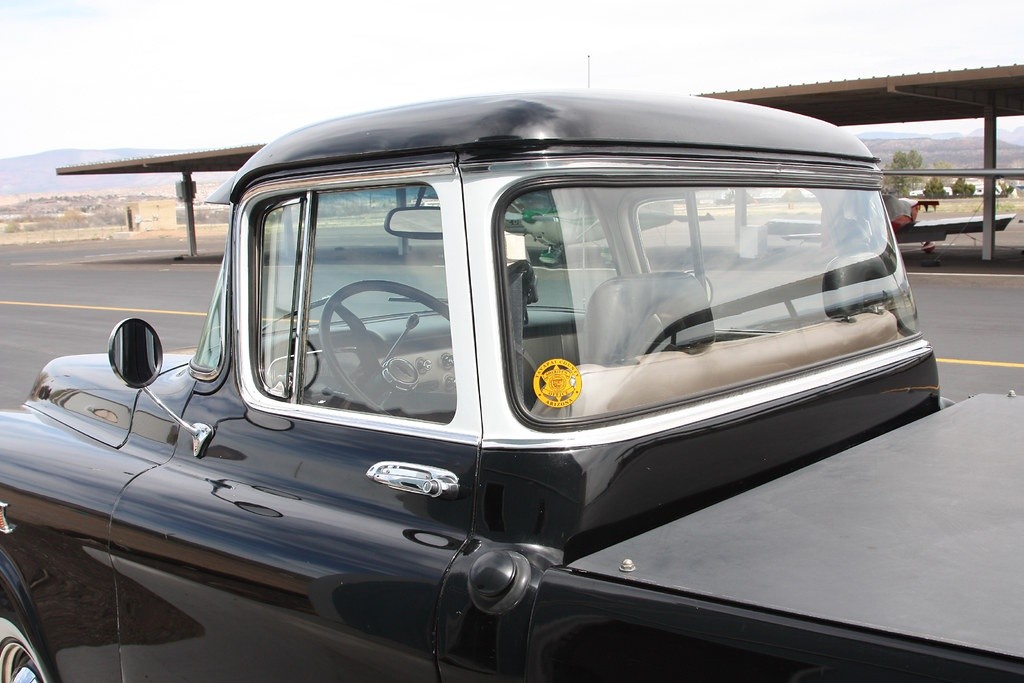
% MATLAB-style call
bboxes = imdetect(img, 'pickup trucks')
[0,86,1021,682]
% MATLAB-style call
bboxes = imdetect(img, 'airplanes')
[768,187,1014,263]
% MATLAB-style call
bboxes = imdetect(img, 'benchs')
[532,252,897,418]
